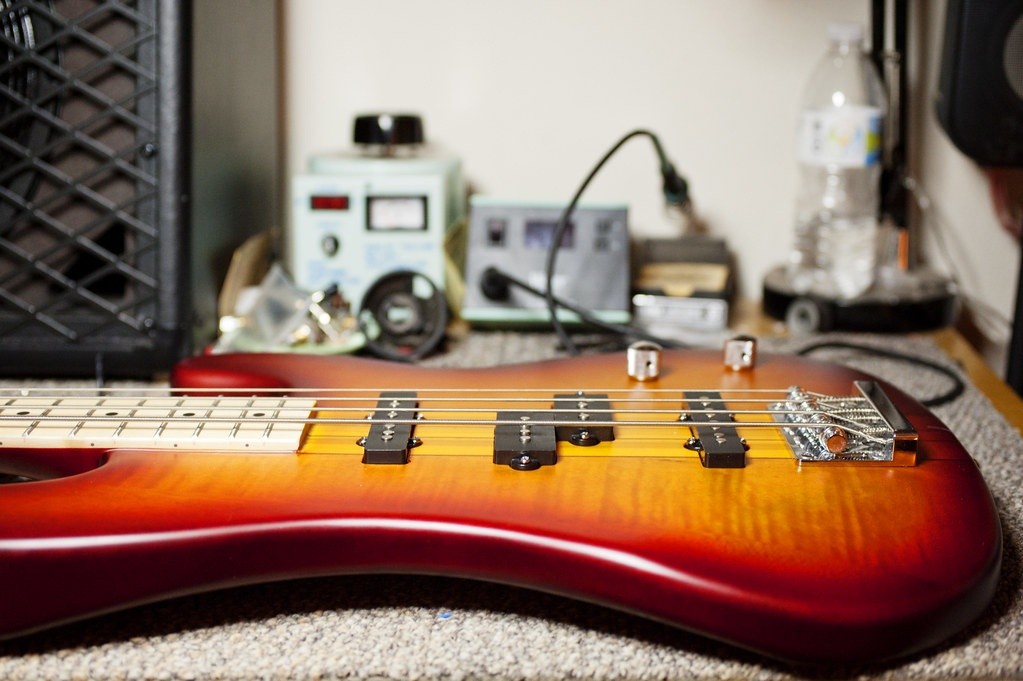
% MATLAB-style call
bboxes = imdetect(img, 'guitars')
[0,346,1004,678]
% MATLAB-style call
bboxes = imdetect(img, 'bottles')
[785,22,890,299]
